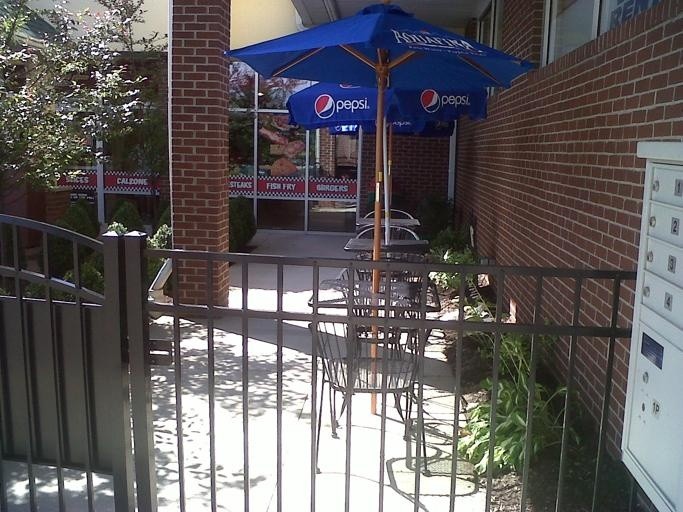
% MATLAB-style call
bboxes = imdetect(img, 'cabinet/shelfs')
[620,140,683,512]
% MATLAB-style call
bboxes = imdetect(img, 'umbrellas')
[284,81,489,261]
[323,122,457,210]
[219,4,542,415]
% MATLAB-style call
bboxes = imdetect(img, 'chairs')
[305,209,442,478]
